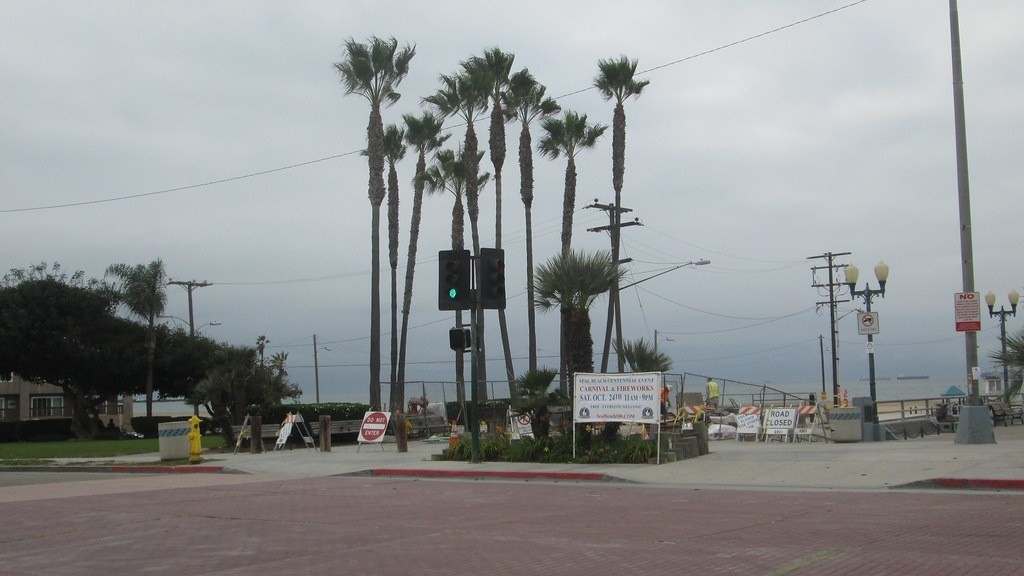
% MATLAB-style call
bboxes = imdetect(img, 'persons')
[660,384,674,416]
[705,378,721,412]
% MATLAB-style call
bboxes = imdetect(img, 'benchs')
[988,403,1024,426]
[229,415,454,452]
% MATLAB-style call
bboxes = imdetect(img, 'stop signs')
[357,411,392,443]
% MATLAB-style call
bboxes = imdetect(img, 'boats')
[859,377,892,380]
[897,375,930,379]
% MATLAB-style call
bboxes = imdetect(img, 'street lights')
[984,290,1020,403]
[313,334,332,402]
[845,259,889,423]
[189,320,222,336]
[601,260,711,373]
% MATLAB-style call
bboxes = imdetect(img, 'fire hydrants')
[188,415,204,465]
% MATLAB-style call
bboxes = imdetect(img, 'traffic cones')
[449,419,458,447]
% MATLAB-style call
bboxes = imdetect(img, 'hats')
[666,384,674,392]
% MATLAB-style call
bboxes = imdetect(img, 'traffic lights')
[481,248,506,310]
[437,250,470,310]
